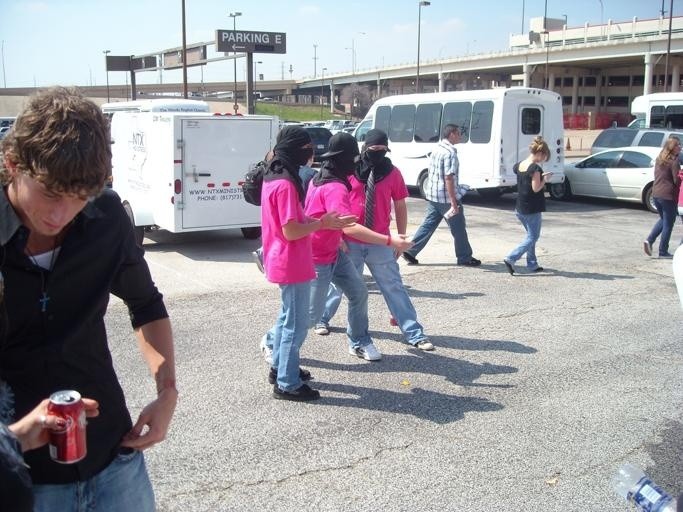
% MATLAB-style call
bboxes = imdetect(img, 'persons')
[260,132,415,364]
[0,87,178,511]
[314,129,434,351]
[643,137,683,260]
[260,124,360,401]
[502,136,553,275]
[403,124,483,267]
[251,143,319,272]
[0,382,100,512]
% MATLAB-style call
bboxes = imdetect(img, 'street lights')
[254,61,263,111]
[321,68,327,120]
[226,12,241,114]
[414,1,431,92]
[538,30,549,90]
[349,31,366,76]
[562,14,568,22]
[102,50,111,103]
[345,47,358,75]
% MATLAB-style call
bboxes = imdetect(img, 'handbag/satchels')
[242,163,267,206]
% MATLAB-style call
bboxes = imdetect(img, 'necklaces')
[26,235,58,312]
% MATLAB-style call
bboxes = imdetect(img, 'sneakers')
[458,257,481,266]
[274,383,320,400]
[268,367,311,384]
[260,335,275,364]
[643,240,651,256]
[659,252,673,258]
[253,250,264,273]
[415,339,434,350]
[503,260,513,276]
[349,343,382,361]
[313,322,329,335]
[399,251,419,265]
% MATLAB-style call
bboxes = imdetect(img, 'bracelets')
[387,235,391,246]
[320,218,324,230]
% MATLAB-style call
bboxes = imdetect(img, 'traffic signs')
[215,29,287,56]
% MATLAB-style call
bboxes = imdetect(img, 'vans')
[591,127,683,156]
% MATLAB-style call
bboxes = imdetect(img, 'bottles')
[610,461,677,511]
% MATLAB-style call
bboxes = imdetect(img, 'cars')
[135,90,151,96]
[256,96,281,104]
[187,91,204,98]
[543,146,683,214]
[280,119,361,166]
[0,116,18,138]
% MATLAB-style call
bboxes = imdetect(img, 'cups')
[443,207,456,220]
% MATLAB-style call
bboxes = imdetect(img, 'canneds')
[47,389,88,465]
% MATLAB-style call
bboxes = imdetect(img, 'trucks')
[98,99,210,112]
[352,86,566,201]
[627,90,682,132]
[102,112,280,245]
